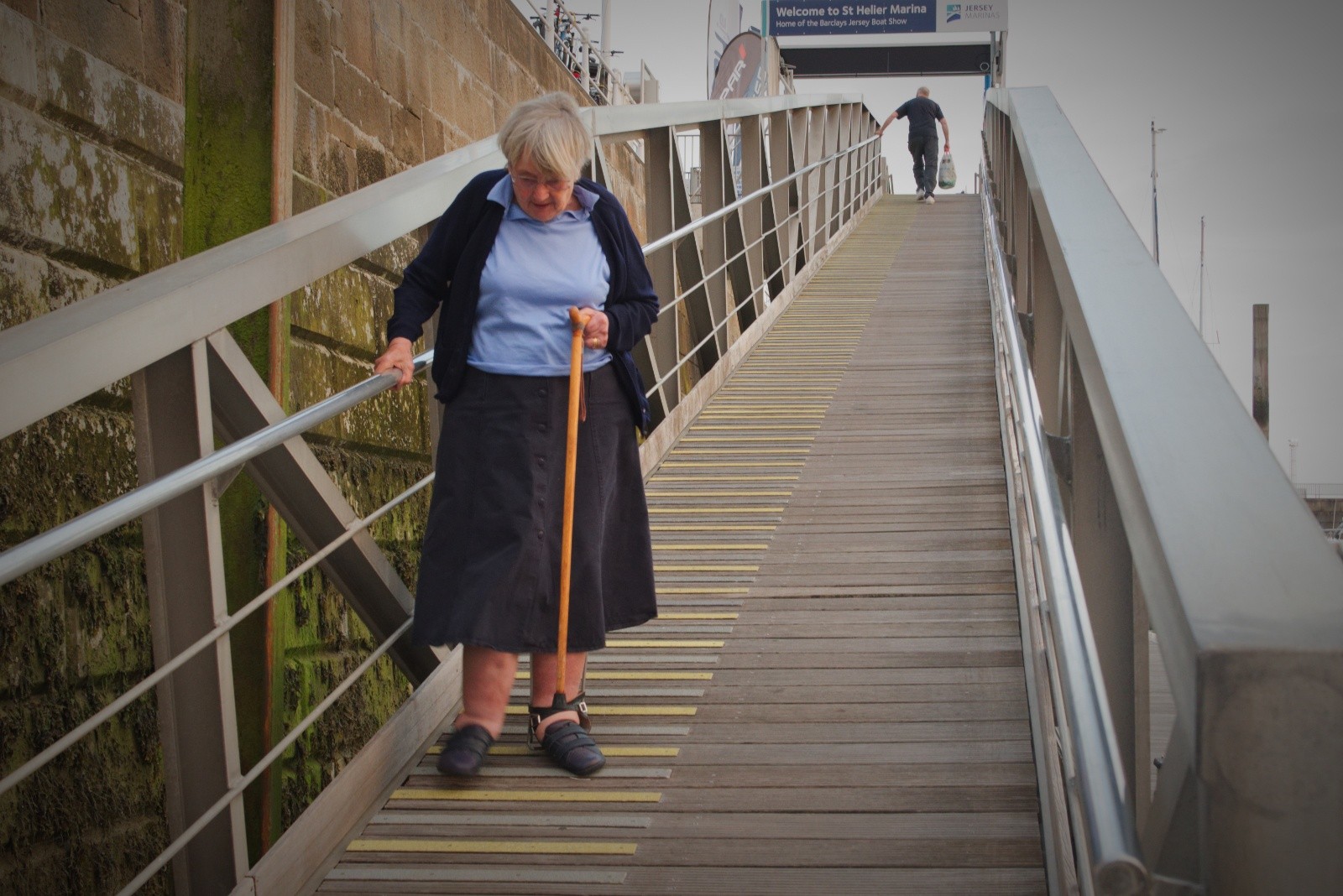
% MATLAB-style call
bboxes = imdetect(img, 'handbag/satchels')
[938,145,956,189]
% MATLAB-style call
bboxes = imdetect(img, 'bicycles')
[530,0,625,103]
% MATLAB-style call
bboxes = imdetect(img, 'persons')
[874,86,950,205]
[375,92,659,780]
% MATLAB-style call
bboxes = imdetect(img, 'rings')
[593,337,599,348]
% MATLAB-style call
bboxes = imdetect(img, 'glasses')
[509,162,572,191]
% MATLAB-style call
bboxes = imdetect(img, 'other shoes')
[916,188,924,201]
[528,691,601,772]
[926,195,934,205]
[438,722,492,775]
